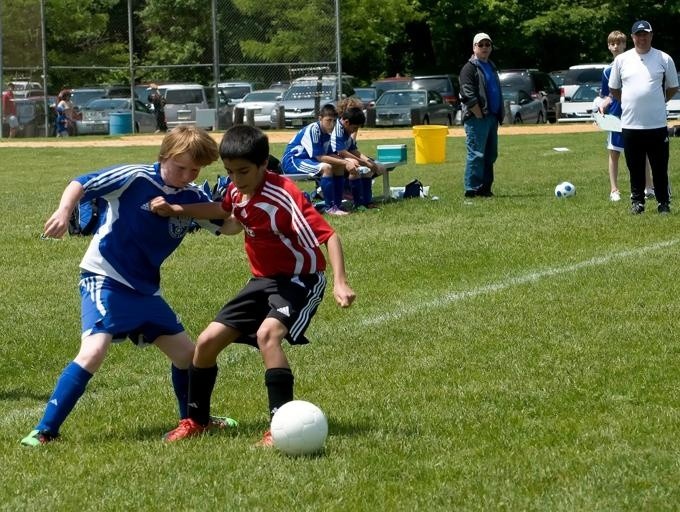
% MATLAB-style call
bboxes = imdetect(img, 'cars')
[354,87,383,110]
[367,89,454,126]
[454,88,546,125]
[1,80,288,136]
[566,80,602,120]
[372,75,413,92]
[665,73,680,121]
[548,69,566,87]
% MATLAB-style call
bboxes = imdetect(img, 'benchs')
[276,161,402,201]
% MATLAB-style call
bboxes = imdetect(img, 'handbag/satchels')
[190,175,232,231]
[68,198,108,235]
[403,180,420,198]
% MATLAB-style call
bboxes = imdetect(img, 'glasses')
[476,42,491,47]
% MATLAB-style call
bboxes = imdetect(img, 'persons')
[592,29,655,201]
[458,31,504,197]
[280,96,386,216]
[149,124,357,449]
[148,83,171,135]
[608,19,678,213]
[50,87,83,136]
[20,123,239,447]
[3,82,19,139]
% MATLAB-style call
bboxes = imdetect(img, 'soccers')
[555,182,576,198]
[270,400,328,455]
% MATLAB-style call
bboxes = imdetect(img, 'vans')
[561,64,609,113]
[408,73,460,112]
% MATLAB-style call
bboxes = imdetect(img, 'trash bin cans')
[111,111,133,136]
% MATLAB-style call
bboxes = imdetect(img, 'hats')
[473,32,493,44]
[632,20,652,34]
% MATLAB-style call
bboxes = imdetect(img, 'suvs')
[270,65,355,127]
[498,67,562,124]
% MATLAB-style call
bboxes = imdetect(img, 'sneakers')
[162,415,237,443]
[325,193,379,216]
[610,186,671,215]
[254,430,274,448]
[21,429,49,447]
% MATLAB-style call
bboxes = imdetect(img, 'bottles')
[357,165,371,175]
